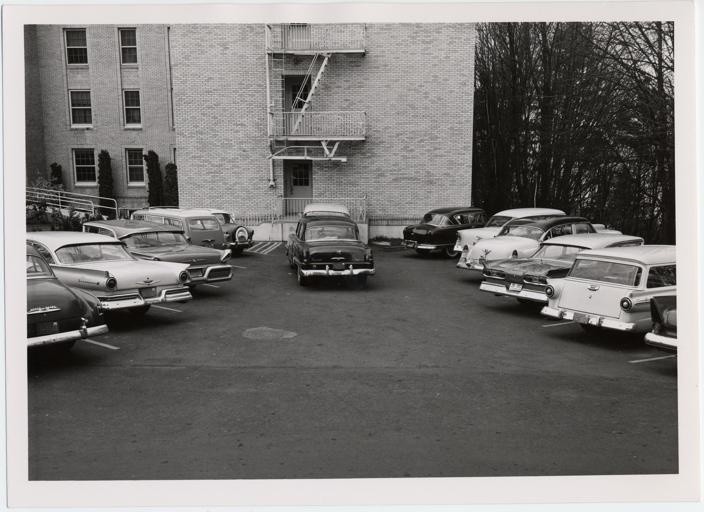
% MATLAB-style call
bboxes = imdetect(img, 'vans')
[130,211,228,260]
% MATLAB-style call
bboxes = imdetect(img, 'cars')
[477,234,637,304]
[81,220,232,287]
[455,208,566,260]
[455,215,623,271]
[30,231,195,320]
[27,245,108,371]
[453,206,565,252]
[542,245,676,335]
[285,201,377,287]
[643,292,678,351]
[200,210,253,258]
[403,205,489,260]
